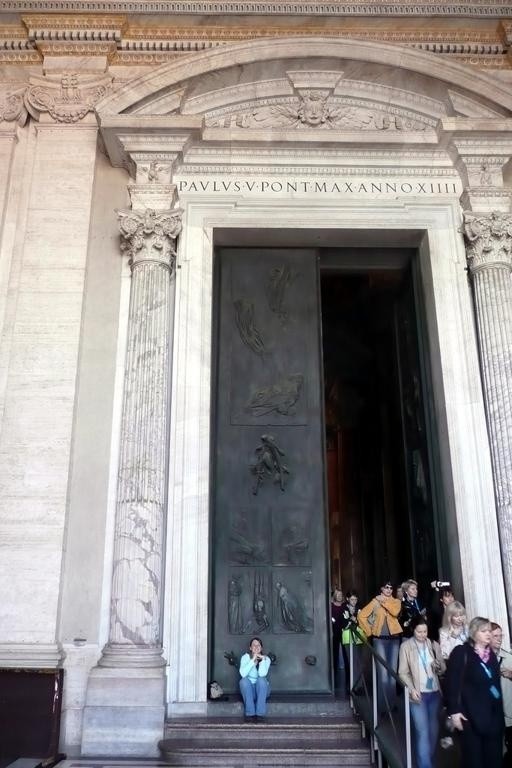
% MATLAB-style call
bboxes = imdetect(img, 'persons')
[249,584,270,634]
[289,90,337,131]
[274,580,310,633]
[329,577,511,768]
[228,572,247,634]
[238,637,274,724]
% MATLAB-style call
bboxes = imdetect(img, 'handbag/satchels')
[438,706,458,736]
[341,627,367,646]
[209,681,224,700]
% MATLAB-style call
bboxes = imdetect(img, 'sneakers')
[245,715,267,725]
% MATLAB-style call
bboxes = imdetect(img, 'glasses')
[385,586,392,590]
